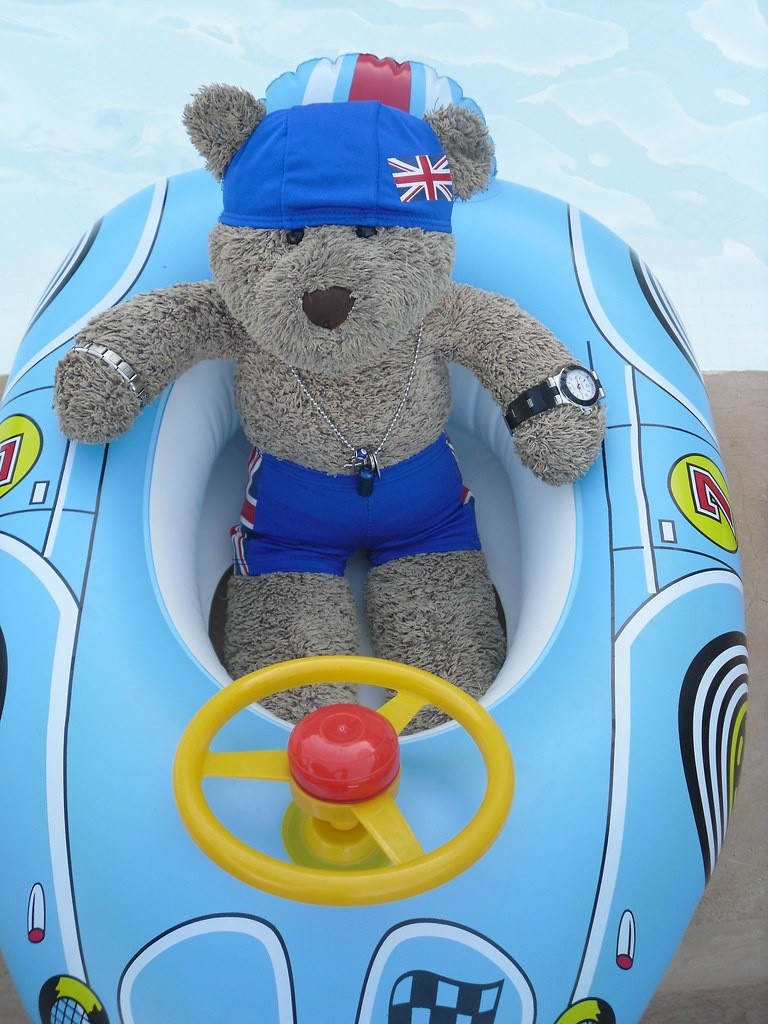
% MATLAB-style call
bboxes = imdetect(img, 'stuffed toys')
[54,85,606,734]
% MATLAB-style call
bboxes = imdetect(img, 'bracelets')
[67,341,149,408]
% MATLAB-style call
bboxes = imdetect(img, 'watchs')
[502,365,605,435]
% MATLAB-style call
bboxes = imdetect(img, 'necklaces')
[287,317,425,477]
